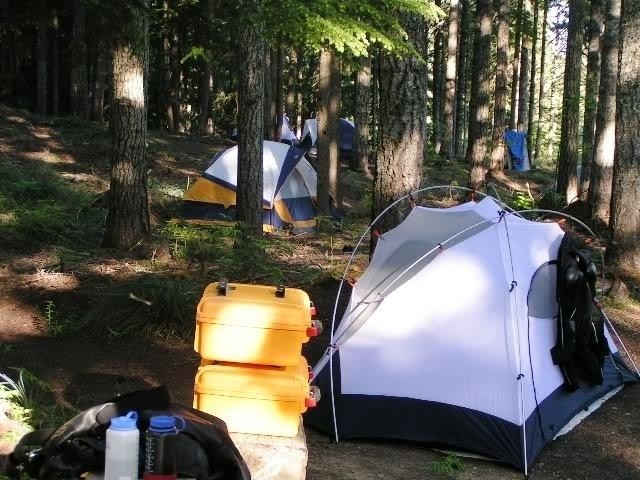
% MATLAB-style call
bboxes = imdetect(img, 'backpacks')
[5,385,251,480]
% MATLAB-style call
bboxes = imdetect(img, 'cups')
[103,410,185,480]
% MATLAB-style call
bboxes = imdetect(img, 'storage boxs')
[193,356,311,439]
[193,278,311,365]
[226,417,309,480]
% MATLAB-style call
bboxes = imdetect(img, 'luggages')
[193,280,323,435]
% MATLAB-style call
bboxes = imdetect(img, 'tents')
[303,186,640,478]
[230,111,355,159]
[169,139,344,237]
